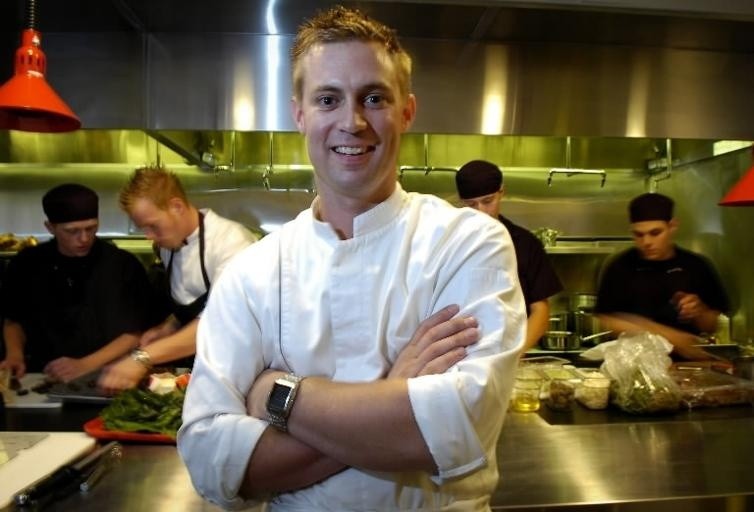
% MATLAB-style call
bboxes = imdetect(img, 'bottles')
[715,315,731,345]
[733,313,745,341]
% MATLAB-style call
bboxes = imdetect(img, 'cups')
[582,377,610,410]
[547,377,577,412]
[511,372,542,410]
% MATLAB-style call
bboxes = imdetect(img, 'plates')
[83,412,178,444]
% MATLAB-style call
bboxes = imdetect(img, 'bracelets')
[129,346,155,371]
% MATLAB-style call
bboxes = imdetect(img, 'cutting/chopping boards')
[5,370,66,410]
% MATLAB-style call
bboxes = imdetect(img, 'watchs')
[265,372,308,435]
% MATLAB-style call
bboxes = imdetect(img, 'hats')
[630,194,673,224]
[42,183,98,223]
[456,160,502,199]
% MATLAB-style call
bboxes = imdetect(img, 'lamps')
[0,0,83,137]
[717,158,754,208]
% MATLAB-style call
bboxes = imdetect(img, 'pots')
[541,329,615,351]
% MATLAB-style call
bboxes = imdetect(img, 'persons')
[176,7,528,511]
[455,159,565,359]
[0,183,154,386]
[97,166,261,396]
[592,193,730,363]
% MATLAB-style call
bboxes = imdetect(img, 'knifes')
[11,439,121,507]
